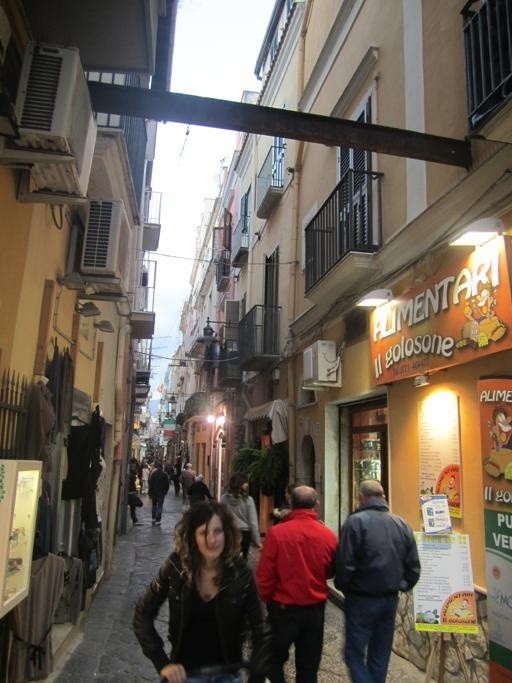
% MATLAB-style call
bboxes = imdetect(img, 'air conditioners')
[12,39,134,300]
[302,339,337,384]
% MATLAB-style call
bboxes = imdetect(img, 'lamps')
[356,288,393,307]
[74,302,115,333]
[449,217,505,247]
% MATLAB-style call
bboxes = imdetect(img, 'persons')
[222,471,265,561]
[140,462,150,495]
[327,479,422,683]
[127,464,138,523]
[173,458,182,495]
[149,461,170,526]
[256,484,339,683]
[187,472,216,507]
[270,478,292,523]
[178,462,197,507]
[132,497,273,682]
